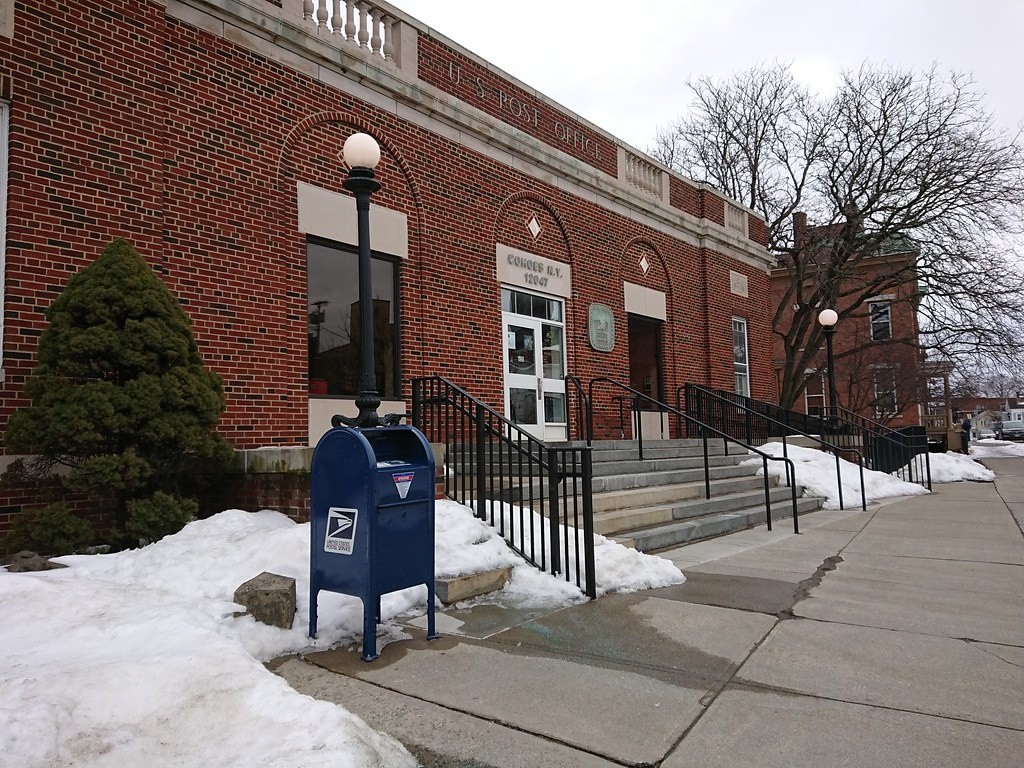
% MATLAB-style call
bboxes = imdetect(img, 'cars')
[996,421,1024,441]
[977,429,996,440]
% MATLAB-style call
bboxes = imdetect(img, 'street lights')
[329,133,404,429]
[818,308,851,436]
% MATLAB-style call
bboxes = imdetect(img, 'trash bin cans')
[928,440,944,453]
[309,426,441,662]
